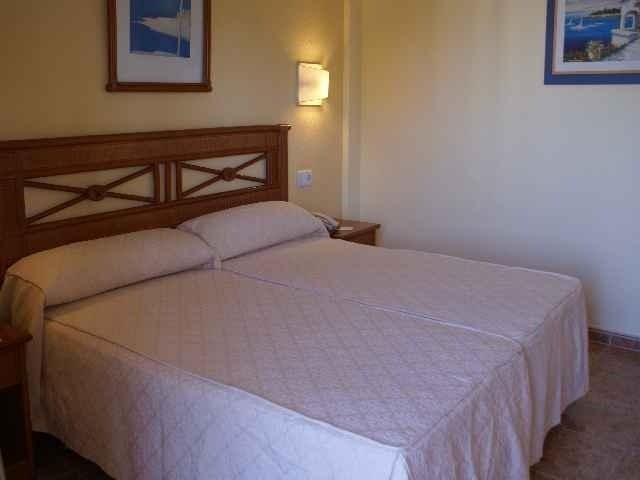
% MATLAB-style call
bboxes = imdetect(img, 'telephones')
[312,212,339,231]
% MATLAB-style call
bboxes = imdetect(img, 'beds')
[2,124,590,480]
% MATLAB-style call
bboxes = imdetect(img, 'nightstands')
[330,218,381,247]
[0,326,34,480]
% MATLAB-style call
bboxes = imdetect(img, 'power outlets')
[296,169,314,187]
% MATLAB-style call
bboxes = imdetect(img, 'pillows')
[177,201,330,268]
[4,228,220,308]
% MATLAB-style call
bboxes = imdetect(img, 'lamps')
[297,61,330,107]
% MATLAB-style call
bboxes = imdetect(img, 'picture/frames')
[107,1,214,92]
[544,1,640,85]
